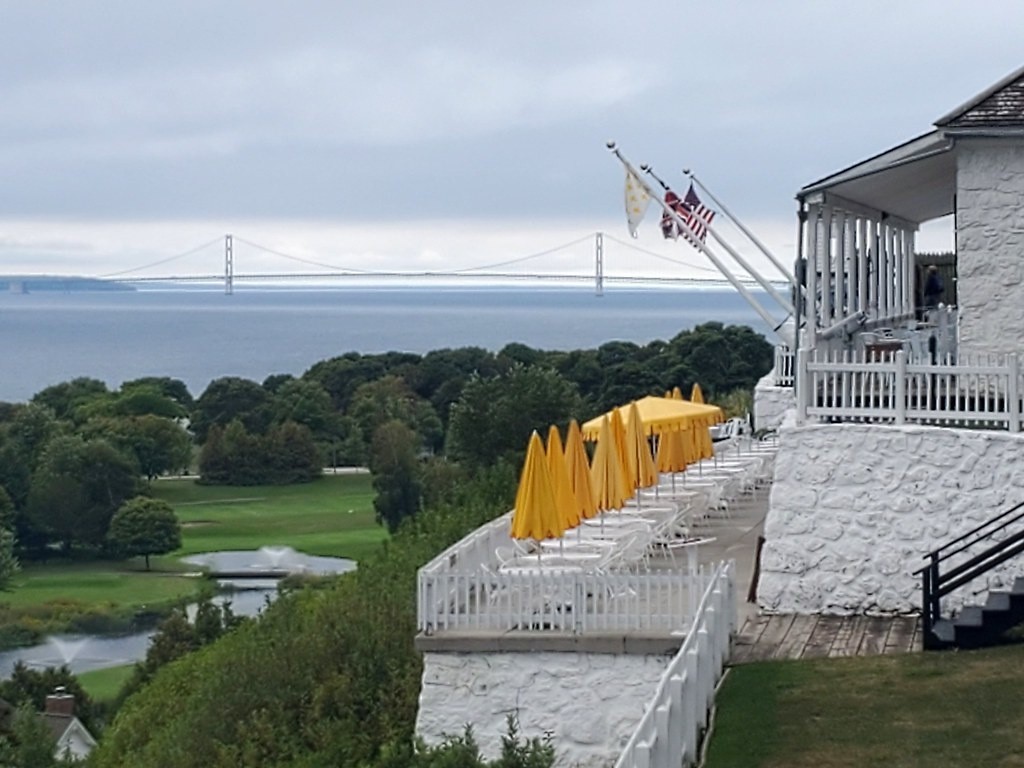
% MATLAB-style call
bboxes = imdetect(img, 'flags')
[677,185,716,253]
[624,173,650,239]
[661,191,692,241]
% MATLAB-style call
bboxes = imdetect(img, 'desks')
[866,339,905,363]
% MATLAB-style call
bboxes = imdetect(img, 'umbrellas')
[580,396,724,501]
[510,400,656,565]
[655,382,715,499]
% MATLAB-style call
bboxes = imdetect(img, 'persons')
[923,265,945,310]
[914,263,924,321]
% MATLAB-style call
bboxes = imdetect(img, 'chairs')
[859,308,958,388]
[478,434,781,605]
